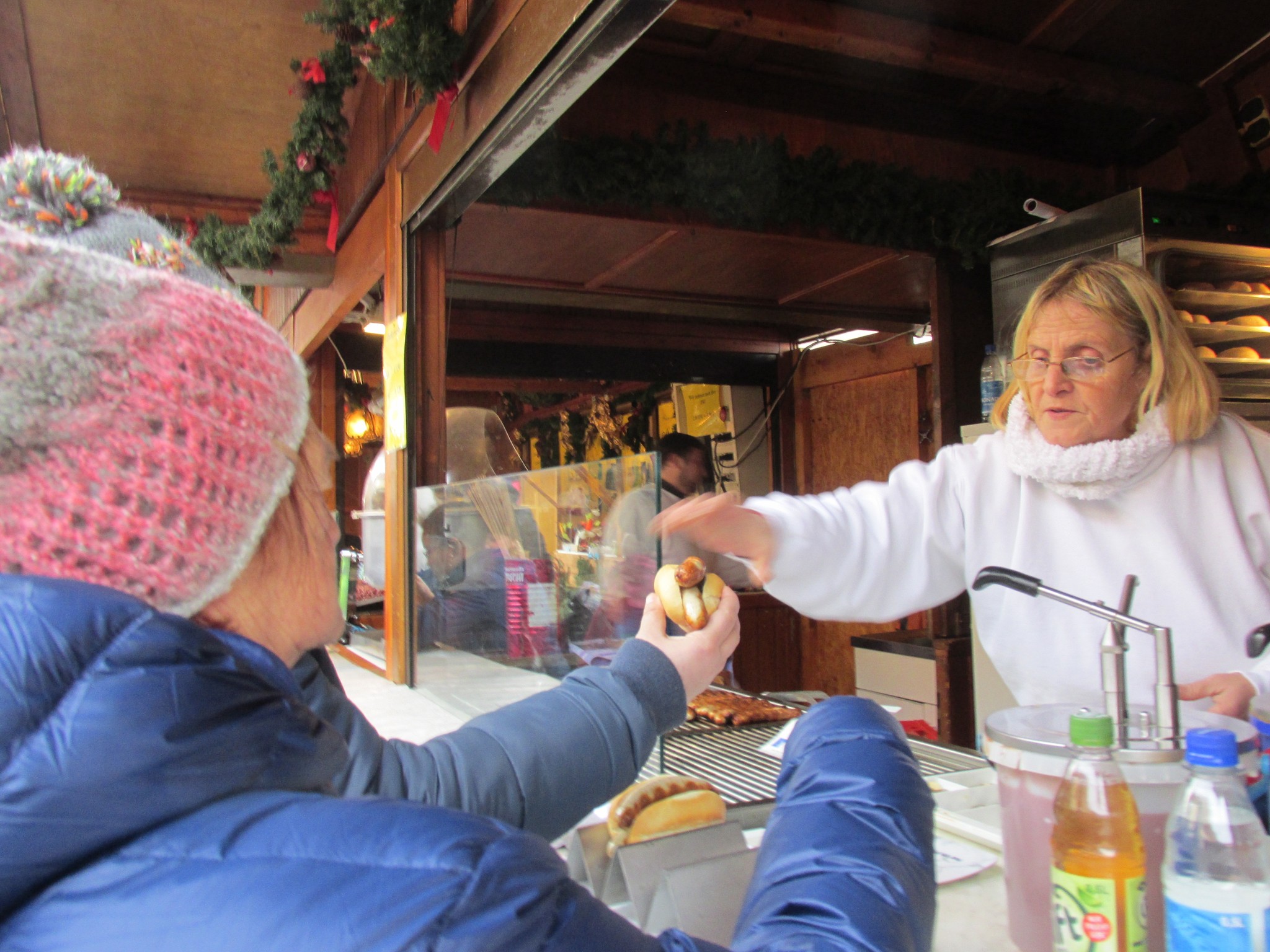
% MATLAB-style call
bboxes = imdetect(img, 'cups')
[503,558,563,657]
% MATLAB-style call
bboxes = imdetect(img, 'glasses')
[421,545,445,558]
[1006,337,1149,380]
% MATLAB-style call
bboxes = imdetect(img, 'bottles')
[1051,713,1147,952]
[1161,726,1270,952]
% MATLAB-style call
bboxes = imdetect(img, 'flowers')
[125,0,463,286]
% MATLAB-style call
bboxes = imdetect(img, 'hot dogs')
[605,775,727,859]
[652,555,727,634]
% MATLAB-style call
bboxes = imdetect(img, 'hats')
[2,148,309,621]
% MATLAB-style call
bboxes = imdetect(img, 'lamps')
[363,301,386,335]
[340,369,384,458]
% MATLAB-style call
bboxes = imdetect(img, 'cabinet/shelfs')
[851,628,940,734]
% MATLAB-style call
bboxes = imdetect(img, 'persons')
[414,500,574,681]
[600,431,766,693]
[644,254,1270,728]
[0,140,746,845]
[0,208,941,951]
